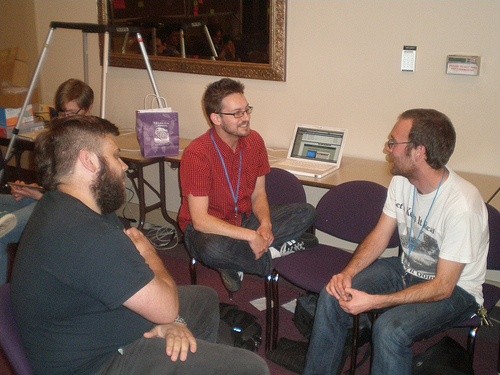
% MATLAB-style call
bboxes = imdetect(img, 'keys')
[478,305,493,327]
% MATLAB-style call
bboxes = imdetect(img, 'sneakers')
[271,232,319,257]
[219,268,243,292]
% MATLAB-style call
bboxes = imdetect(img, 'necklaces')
[401,169,445,289]
[209,127,243,225]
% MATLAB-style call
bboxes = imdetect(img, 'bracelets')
[174,315,187,325]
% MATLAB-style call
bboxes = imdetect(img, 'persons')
[176,79,316,291]
[10,115,270,375]
[0,79,94,288]
[304,108,489,375]
[148,24,242,62]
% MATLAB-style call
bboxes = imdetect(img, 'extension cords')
[130,222,154,230]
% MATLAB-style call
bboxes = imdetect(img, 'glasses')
[388,139,414,149]
[56,106,83,118]
[214,105,252,118]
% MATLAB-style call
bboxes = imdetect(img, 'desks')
[0,120,183,232]
[165,137,500,212]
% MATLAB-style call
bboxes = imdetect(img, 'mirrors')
[96,0,287,83]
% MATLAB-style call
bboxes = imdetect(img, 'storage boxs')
[0,47,40,138]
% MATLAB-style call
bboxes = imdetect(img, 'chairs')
[189,169,500,375]
[0,283,34,375]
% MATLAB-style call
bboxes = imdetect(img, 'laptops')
[269,123,348,178]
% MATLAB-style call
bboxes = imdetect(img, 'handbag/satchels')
[217,302,263,352]
[292,291,374,349]
[135,94,179,158]
[411,335,474,375]
